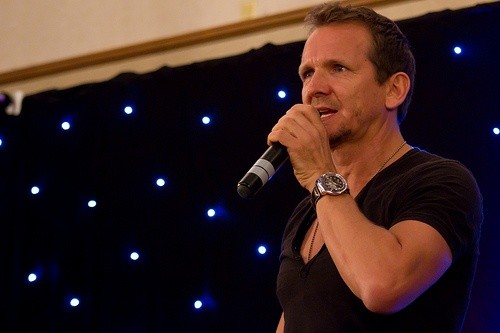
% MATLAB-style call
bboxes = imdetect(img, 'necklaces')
[309,142,407,262]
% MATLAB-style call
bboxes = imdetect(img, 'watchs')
[308,171,349,210]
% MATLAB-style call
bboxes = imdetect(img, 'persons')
[251,3,482,333]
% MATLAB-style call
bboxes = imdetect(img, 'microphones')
[236,140,289,198]
